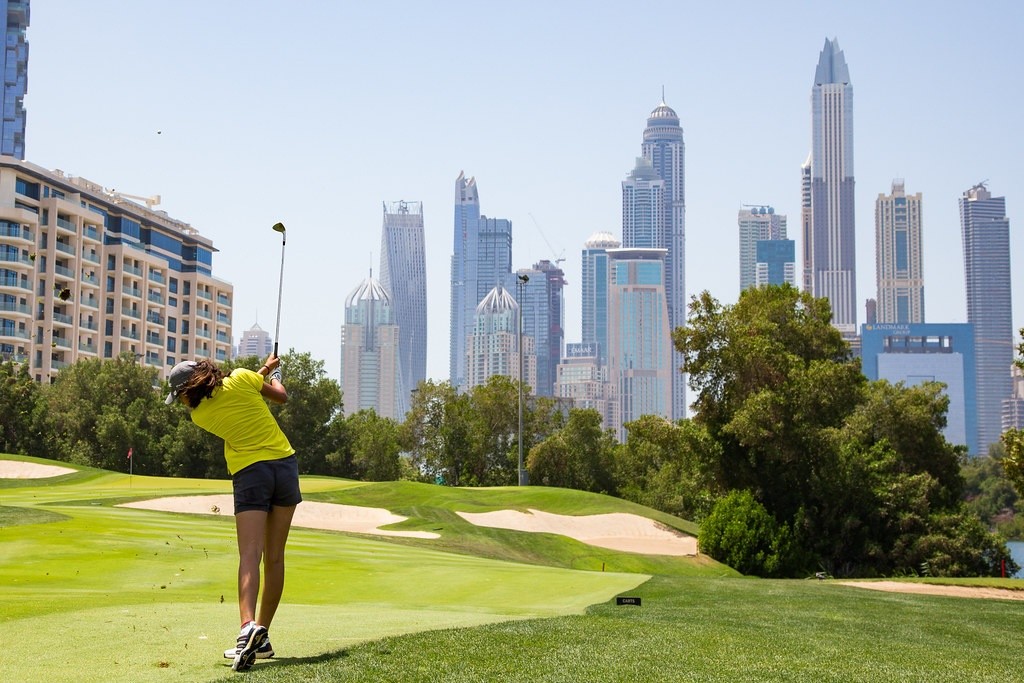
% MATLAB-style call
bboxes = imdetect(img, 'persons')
[165,351,302,674]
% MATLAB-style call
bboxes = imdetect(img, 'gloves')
[268,369,282,385]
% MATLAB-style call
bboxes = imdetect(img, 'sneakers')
[231,620,268,671]
[224,632,274,661]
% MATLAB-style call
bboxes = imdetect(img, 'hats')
[164,360,197,406]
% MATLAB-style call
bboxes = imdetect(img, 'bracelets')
[264,365,271,376]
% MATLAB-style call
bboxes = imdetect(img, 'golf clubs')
[272,219,286,360]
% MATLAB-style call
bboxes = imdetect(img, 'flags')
[127,450,131,458]
